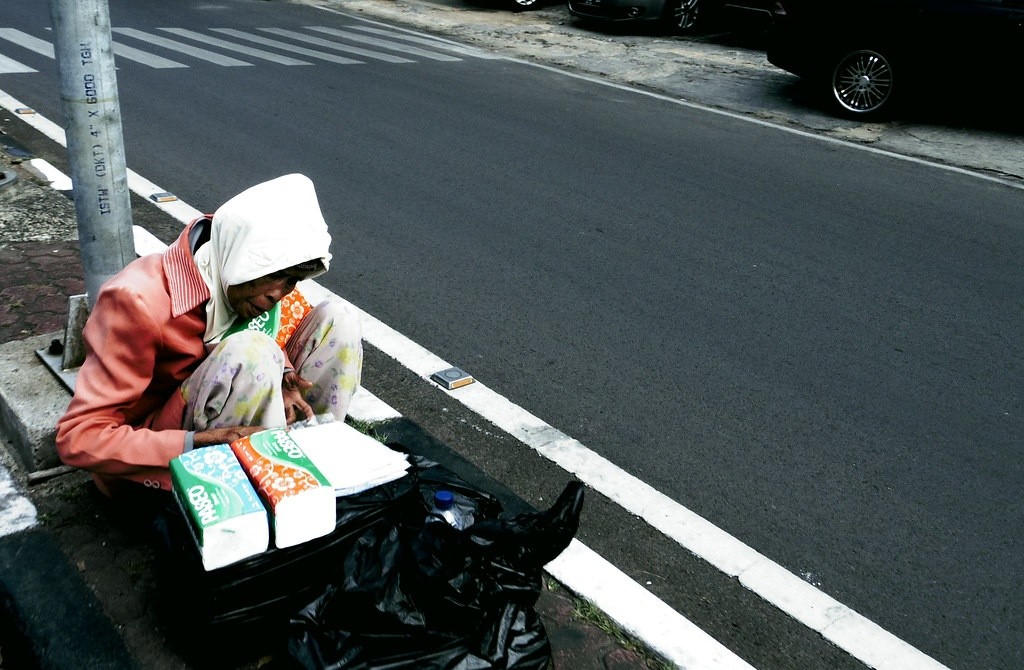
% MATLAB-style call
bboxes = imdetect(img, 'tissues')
[168,424,339,573]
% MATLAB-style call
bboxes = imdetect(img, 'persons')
[51,171,364,500]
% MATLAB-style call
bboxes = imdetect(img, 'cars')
[564,0,765,31]
[765,0,1024,119]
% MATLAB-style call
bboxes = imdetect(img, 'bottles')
[426,491,465,530]
